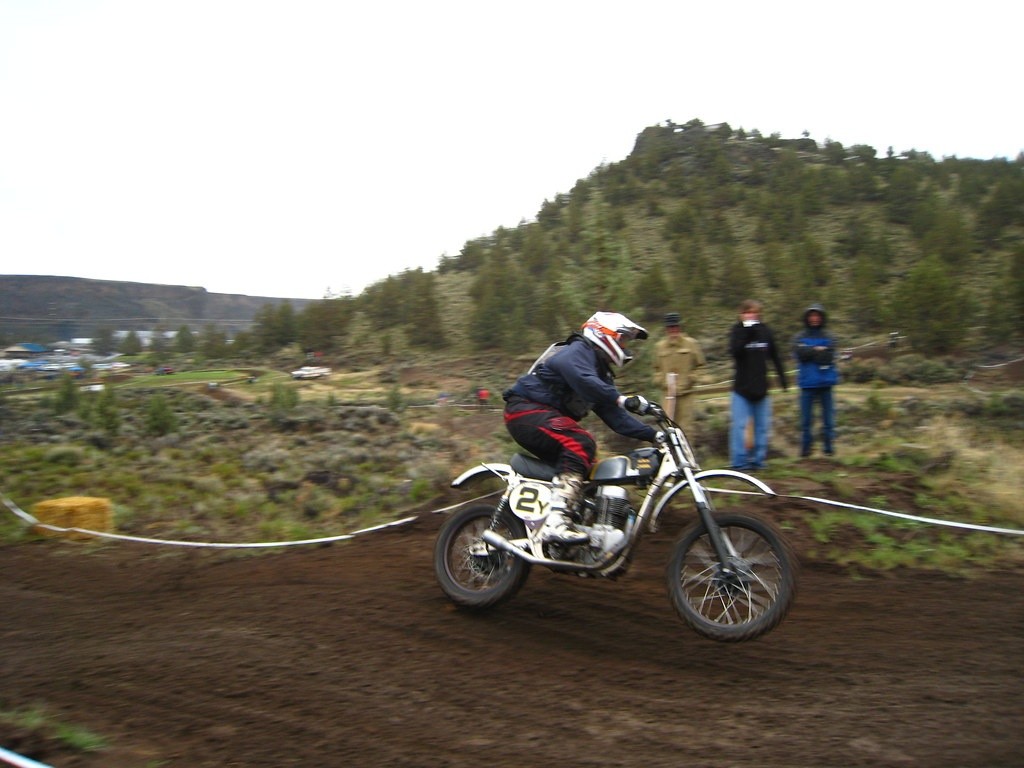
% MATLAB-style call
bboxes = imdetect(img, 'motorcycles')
[432,395,801,643]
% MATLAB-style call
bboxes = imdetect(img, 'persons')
[726,301,790,468]
[793,302,839,458]
[501,310,665,543]
[654,311,707,441]
[477,385,489,412]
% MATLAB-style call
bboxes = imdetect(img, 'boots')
[538,472,591,544]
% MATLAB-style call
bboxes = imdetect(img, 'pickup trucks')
[291,366,331,378]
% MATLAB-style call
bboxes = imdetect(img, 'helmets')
[583,311,649,366]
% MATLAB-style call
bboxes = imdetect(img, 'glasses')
[614,333,631,350]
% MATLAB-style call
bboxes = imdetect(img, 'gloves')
[620,393,649,414]
[653,432,668,444]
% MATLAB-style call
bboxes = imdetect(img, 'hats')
[664,311,680,326]
